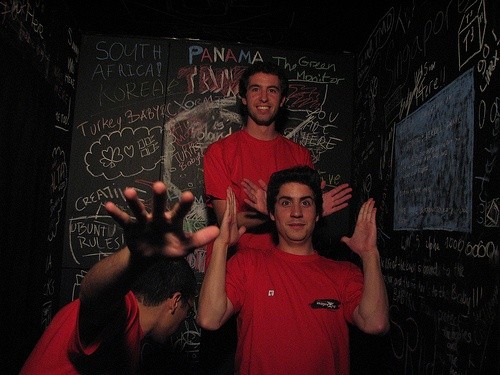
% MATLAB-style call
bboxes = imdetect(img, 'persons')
[16,181,220,374]
[195,165,390,375]
[203,63,352,374]
